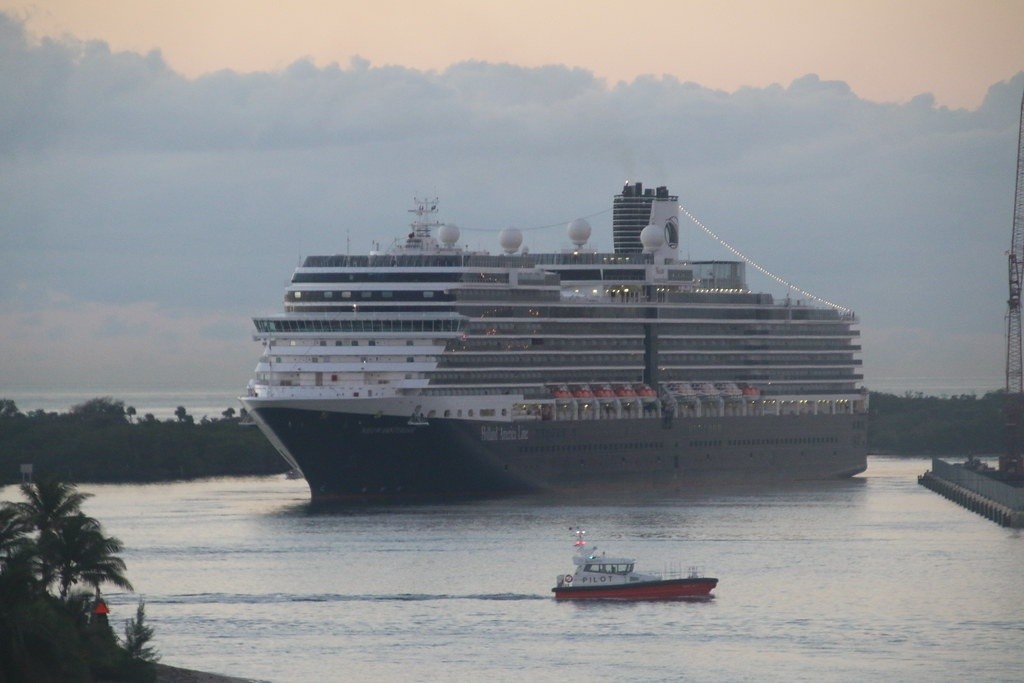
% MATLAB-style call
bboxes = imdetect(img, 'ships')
[238,177,868,505]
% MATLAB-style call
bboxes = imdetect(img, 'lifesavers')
[566,574,572,582]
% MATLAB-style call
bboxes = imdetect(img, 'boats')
[550,550,720,602]
